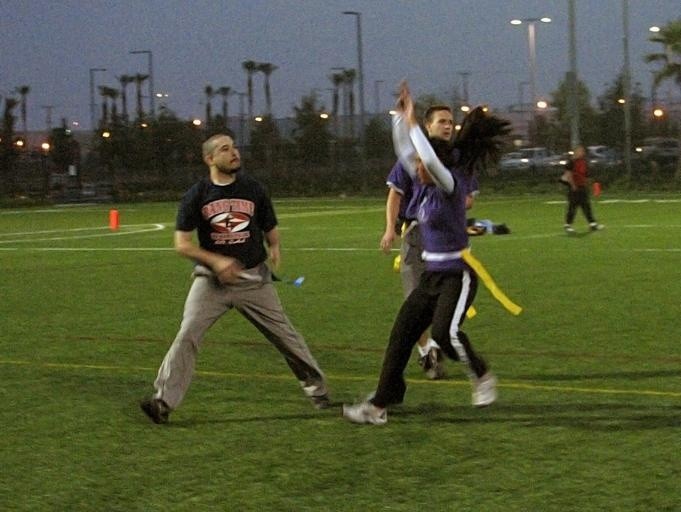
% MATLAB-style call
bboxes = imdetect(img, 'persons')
[559,144,606,236]
[339,77,511,428]
[377,102,477,383]
[135,130,333,426]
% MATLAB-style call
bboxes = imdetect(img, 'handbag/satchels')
[492,224,509,234]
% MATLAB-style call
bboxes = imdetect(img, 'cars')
[500,135,680,178]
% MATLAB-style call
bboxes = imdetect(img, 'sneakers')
[471,373,497,407]
[565,222,605,231]
[419,347,447,379]
[142,399,168,422]
[341,404,387,426]
[310,395,330,409]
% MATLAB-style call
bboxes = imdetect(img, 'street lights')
[645,23,681,131]
[509,15,551,145]
